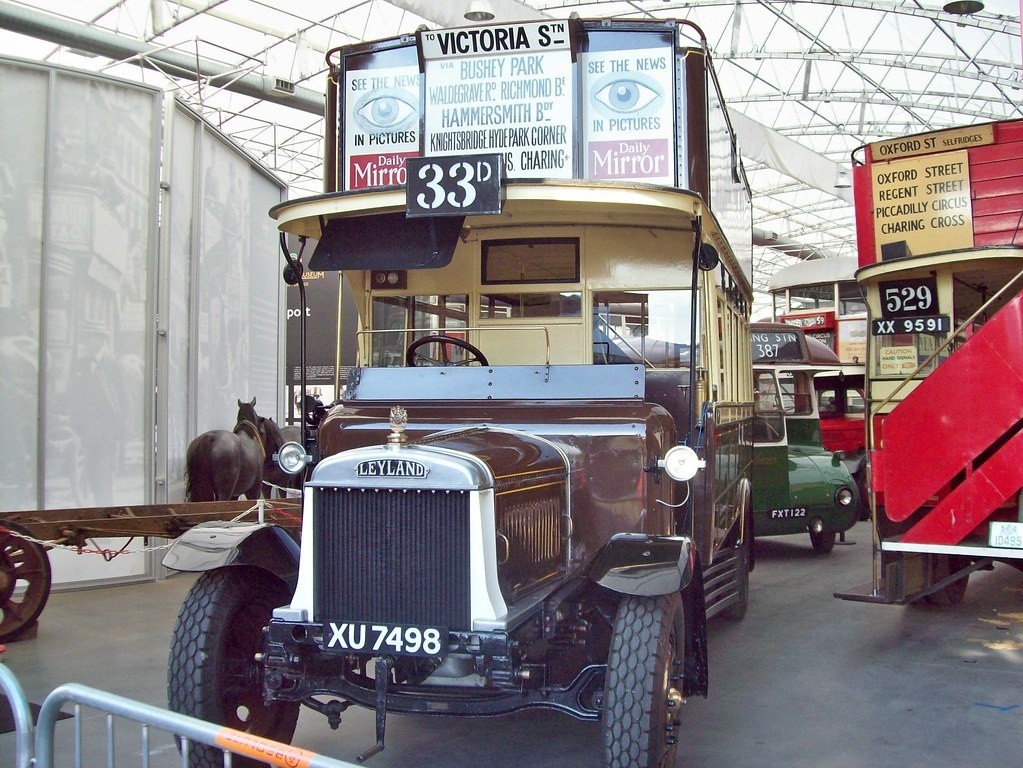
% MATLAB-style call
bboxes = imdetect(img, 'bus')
[166,10,757,768]
[600,323,876,573]
[849,113,1023,613]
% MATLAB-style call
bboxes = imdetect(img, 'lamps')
[832,175,853,188]
[464,0,495,20]
[942,0,985,14]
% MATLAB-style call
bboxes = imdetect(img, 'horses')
[183,397,265,501]
[256,414,303,500]
